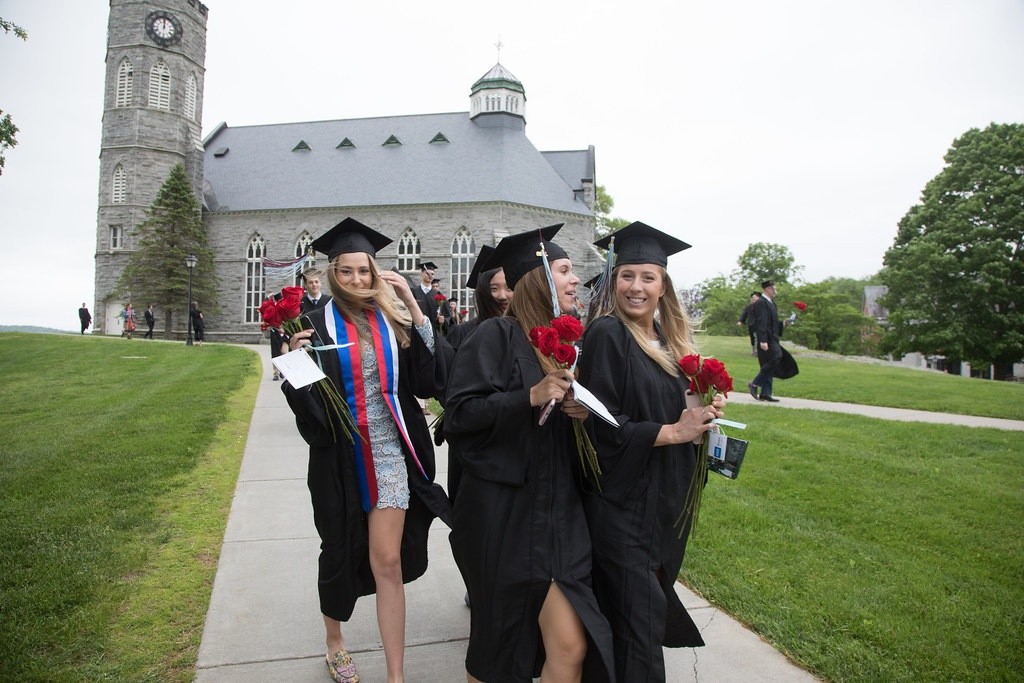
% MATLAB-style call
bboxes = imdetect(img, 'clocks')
[144,11,188,46]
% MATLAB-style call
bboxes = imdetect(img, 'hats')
[584,221,693,309]
[583,267,616,290]
[296,268,322,283]
[262,217,394,287]
[479,223,570,319]
[751,291,761,298]
[761,280,774,288]
[416,262,438,279]
[431,278,440,284]
[465,245,498,289]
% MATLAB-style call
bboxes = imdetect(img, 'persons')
[79,303,91,334]
[576,221,726,683]
[738,292,761,357]
[439,243,516,607]
[750,280,799,401]
[296,267,331,313]
[416,261,463,414]
[281,218,449,683]
[445,222,616,683]
[144,305,155,340]
[123,304,137,339]
[192,302,205,342]
[568,296,587,322]
[270,295,289,380]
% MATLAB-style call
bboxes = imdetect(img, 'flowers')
[530,316,601,492]
[258,287,366,446]
[674,354,733,541]
[790,301,806,321]
[458,310,468,321]
[434,294,446,335]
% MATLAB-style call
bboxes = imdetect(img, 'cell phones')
[539,398,555,426]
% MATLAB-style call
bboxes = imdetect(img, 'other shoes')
[280,372,284,378]
[759,393,780,402]
[273,375,279,380]
[326,650,359,683]
[748,383,758,400]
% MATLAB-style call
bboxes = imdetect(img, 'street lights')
[183,253,199,346]
